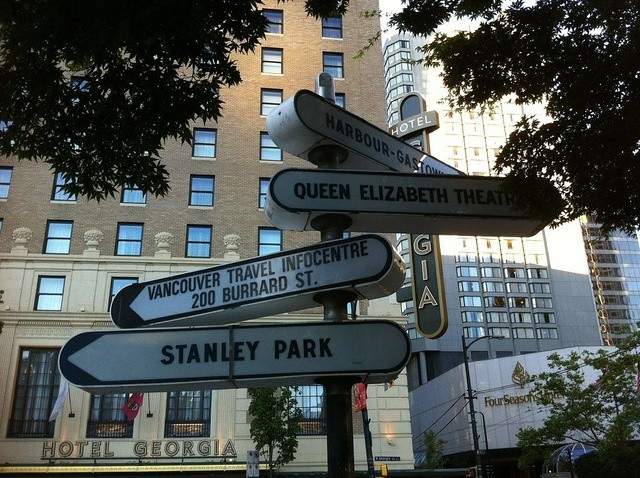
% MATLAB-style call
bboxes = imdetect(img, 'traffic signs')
[109,233,406,328]
[265,167,563,237]
[267,88,467,174]
[58,318,412,395]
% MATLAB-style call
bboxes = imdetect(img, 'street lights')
[461,335,504,477]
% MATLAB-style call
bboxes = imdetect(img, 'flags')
[122,392,144,422]
[49,377,68,422]
